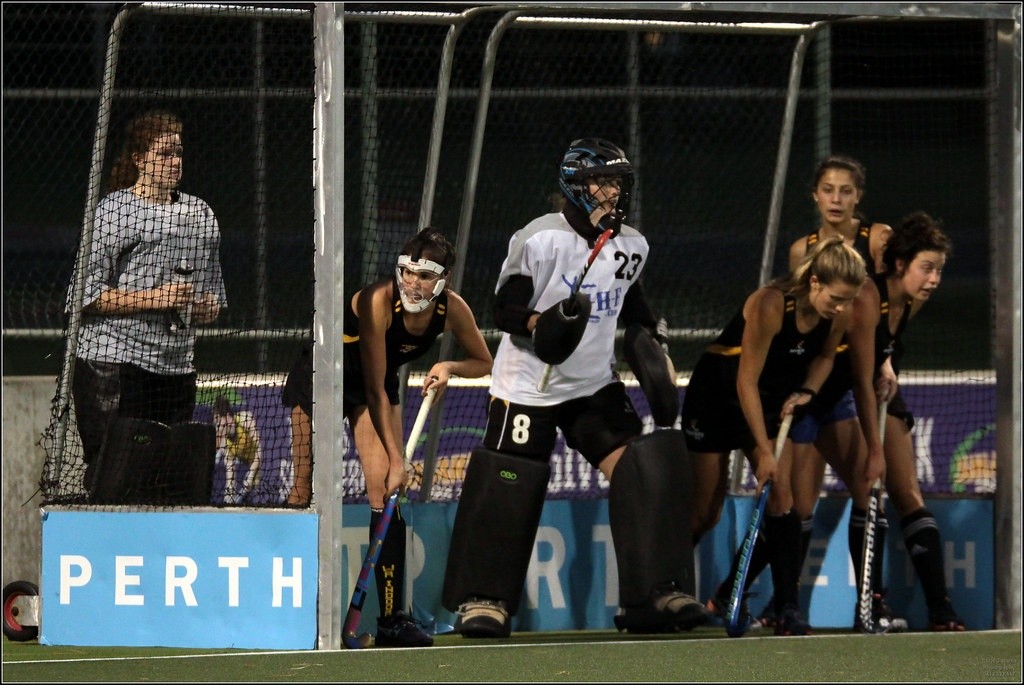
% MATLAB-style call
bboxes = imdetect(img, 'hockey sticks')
[342,375,436,649]
[539,228,615,390]
[724,392,797,643]
[860,379,908,633]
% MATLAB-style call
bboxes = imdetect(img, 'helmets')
[560,137,634,218]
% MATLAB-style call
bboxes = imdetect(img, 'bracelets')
[797,388,817,401]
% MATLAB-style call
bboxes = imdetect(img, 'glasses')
[144,146,183,159]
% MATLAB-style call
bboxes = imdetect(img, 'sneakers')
[927,590,966,632]
[706,580,751,637]
[756,596,812,636]
[375,607,433,647]
[853,596,907,633]
[454,595,512,638]
[614,590,713,634]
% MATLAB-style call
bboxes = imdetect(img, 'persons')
[282,227,494,647]
[442,136,714,633]
[679,150,965,638]
[211,396,263,504]
[63,110,226,485]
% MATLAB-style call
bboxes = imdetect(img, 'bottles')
[165,258,195,335]
